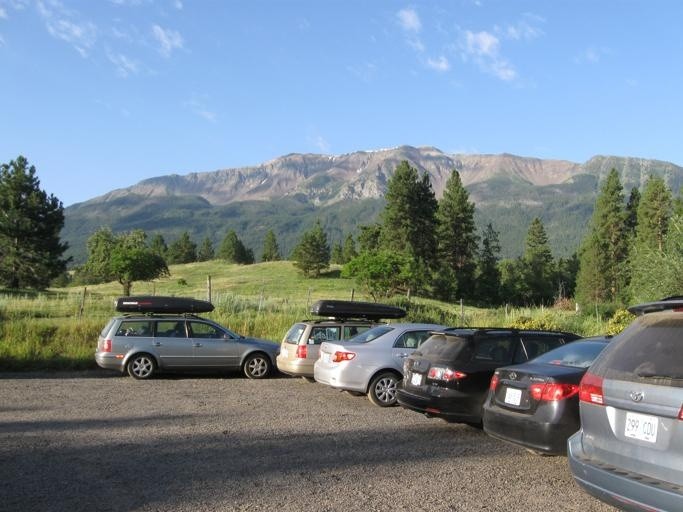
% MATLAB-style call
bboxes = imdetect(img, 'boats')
[112,295,216,315]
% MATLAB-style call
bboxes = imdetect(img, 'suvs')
[95,294,277,380]
